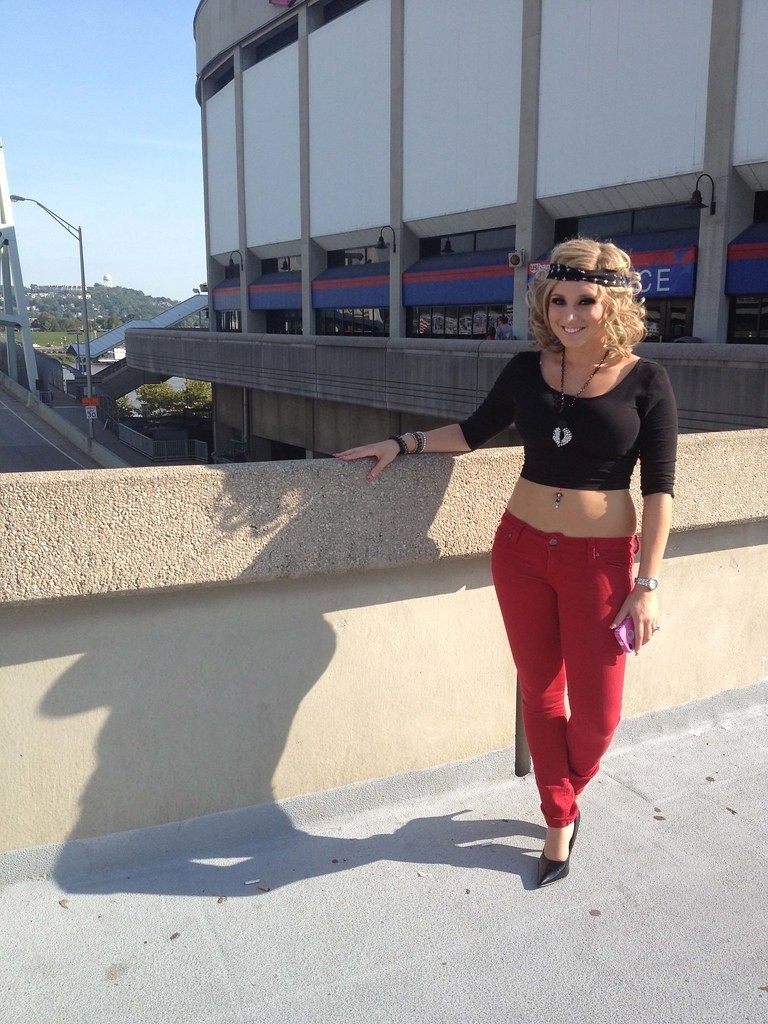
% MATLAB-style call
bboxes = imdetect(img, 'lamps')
[229,251,244,271]
[684,173,716,215]
[281,256,291,271]
[375,226,397,253]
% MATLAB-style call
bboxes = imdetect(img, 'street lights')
[10,195,94,440]
[193,288,201,329]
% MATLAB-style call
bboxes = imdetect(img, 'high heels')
[538,810,580,887]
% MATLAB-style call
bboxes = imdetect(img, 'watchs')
[634,577,659,590]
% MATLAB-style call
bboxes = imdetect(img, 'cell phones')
[614,614,636,653]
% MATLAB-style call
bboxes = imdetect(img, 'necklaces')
[557,348,609,430]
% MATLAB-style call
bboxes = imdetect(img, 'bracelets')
[389,432,426,455]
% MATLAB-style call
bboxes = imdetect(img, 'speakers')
[507,252,523,268]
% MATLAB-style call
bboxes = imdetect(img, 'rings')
[653,626,660,632]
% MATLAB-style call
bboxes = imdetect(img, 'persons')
[332,240,678,888]
[486,317,512,340]
[671,319,681,338]
